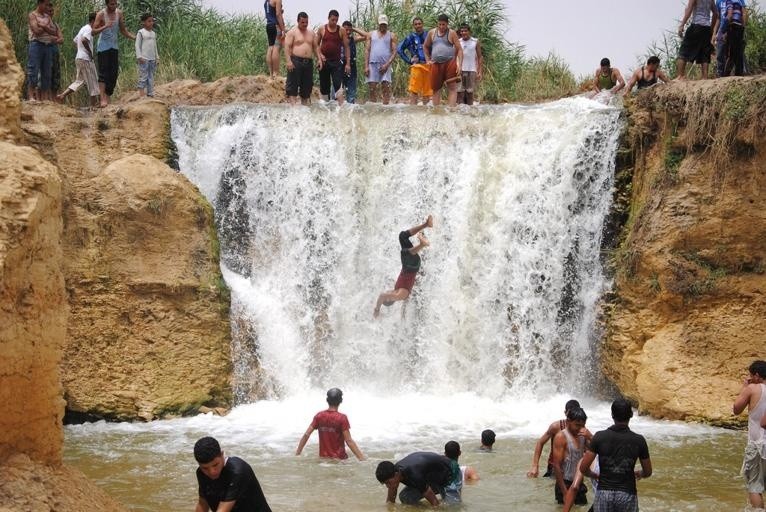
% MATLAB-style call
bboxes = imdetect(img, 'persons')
[563,435,600,512]
[444,441,480,482]
[374,214,433,318]
[733,360,766,510]
[622,56,671,97]
[589,58,625,99]
[552,406,594,506]
[526,400,580,478]
[671,0,749,82]
[375,452,462,509]
[295,388,367,461]
[478,430,496,452]
[26,0,160,110]
[579,398,652,512]
[263,0,484,109]
[194,436,273,512]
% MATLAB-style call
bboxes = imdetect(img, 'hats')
[378,15,388,25]
